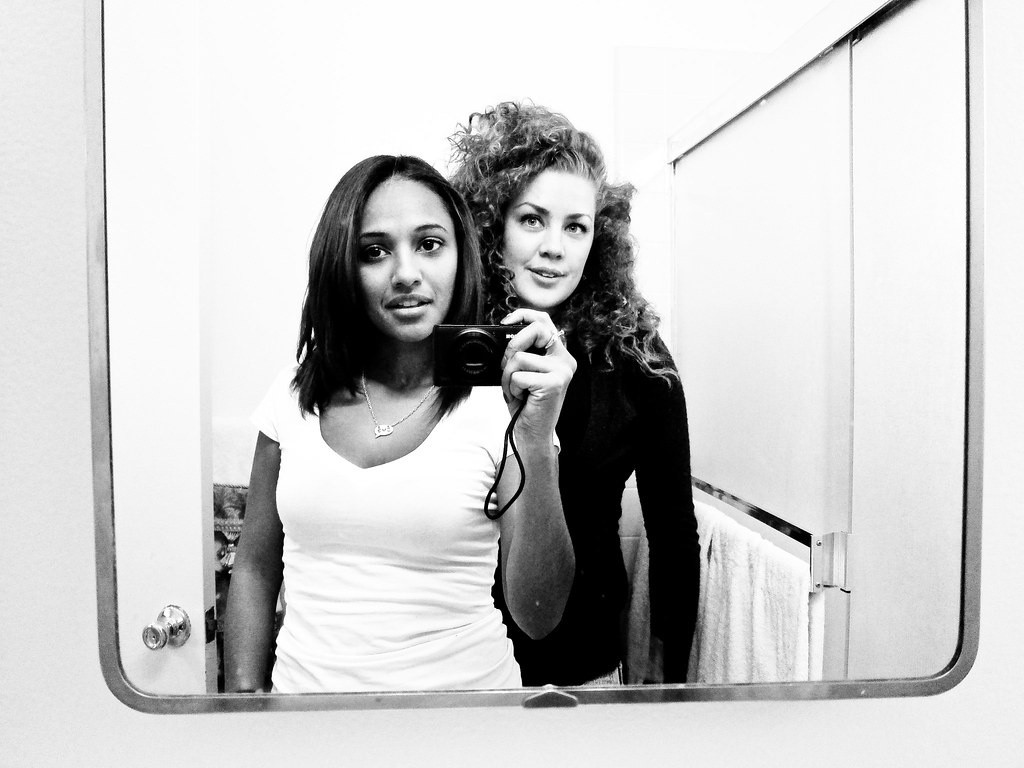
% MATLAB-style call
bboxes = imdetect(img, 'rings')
[544,328,568,352]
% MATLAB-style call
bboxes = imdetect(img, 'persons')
[444,100,702,688]
[223,154,578,694]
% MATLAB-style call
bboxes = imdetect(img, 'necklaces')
[361,374,435,440]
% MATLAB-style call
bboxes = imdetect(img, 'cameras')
[432,323,539,387]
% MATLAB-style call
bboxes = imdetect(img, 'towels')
[626,499,825,682]
